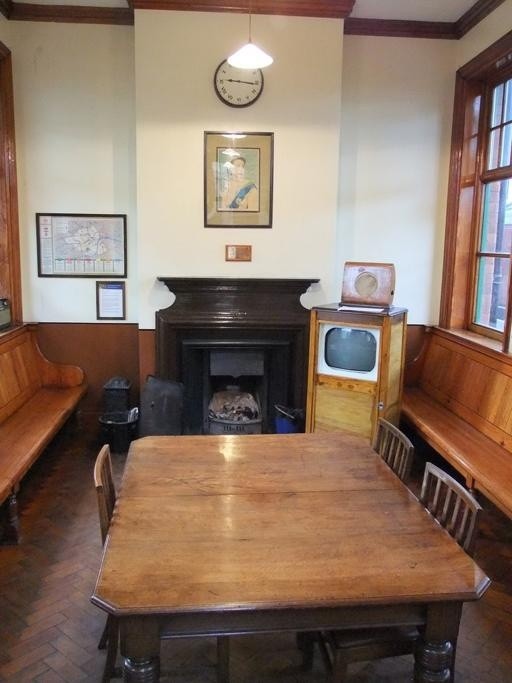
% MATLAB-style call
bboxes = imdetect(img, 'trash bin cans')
[99,412,139,453]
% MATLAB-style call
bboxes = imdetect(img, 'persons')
[220,153,259,211]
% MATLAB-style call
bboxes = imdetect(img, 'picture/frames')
[96,281,126,320]
[38,214,127,278]
[204,132,273,228]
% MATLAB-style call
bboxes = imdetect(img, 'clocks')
[213,59,264,108]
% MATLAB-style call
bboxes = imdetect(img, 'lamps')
[227,0,273,71]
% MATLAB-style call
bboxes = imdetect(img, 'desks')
[89,432,491,683]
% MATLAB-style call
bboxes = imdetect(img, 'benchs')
[0,323,88,547]
[399,324,512,555]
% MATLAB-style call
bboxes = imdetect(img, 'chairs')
[374,417,415,484]
[420,461,483,554]
[94,444,229,683]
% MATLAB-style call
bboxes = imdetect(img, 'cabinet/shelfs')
[305,307,407,447]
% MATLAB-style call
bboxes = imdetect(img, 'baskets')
[209,390,263,434]
